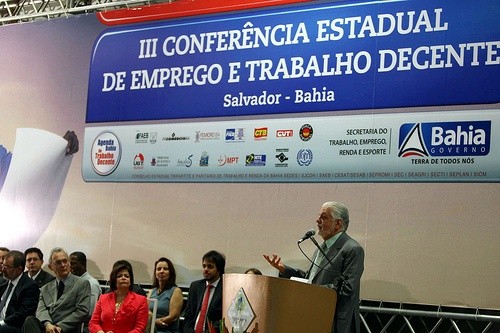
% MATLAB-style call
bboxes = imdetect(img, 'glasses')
[26,257,40,262]
[2,264,14,270]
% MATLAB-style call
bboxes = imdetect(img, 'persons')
[245,268,262,275]
[184,251,226,333]
[24,248,54,288]
[0,248,39,333]
[21,247,91,333]
[69,252,102,324]
[263,202,364,333]
[0,130,79,192]
[147,257,183,333]
[88,260,149,333]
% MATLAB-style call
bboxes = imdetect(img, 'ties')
[57,280,65,301]
[195,284,213,333]
[307,243,327,284]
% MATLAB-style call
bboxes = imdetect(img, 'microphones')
[298,229,315,243]
